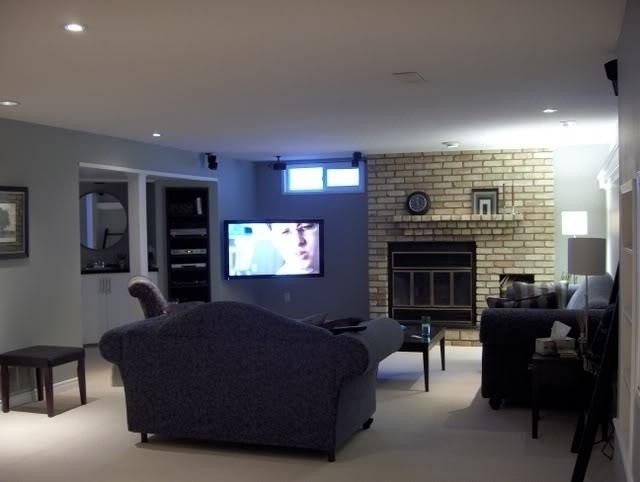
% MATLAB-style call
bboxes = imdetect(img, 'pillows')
[487,292,559,309]
[505,282,569,309]
[161,300,203,317]
[323,316,368,333]
[300,312,331,325]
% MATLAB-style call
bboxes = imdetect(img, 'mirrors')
[79,190,128,251]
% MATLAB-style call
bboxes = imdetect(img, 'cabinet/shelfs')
[611,1,639,482]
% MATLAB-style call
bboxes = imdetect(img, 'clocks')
[406,191,431,215]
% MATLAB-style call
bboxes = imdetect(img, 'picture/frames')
[0,185,29,260]
[472,189,499,215]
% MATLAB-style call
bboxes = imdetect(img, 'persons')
[266,222,320,276]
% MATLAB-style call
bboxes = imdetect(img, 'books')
[560,356,576,358]
[561,350,577,356]
[557,348,574,353]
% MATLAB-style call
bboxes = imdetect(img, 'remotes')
[332,326,366,333]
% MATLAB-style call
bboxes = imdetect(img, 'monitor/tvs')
[223,219,325,278]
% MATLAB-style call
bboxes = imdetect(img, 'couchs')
[97,301,404,463]
[479,273,614,409]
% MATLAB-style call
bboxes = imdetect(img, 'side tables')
[529,349,600,438]
[0,345,85,417]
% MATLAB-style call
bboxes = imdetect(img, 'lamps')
[567,238,606,356]
[562,210,588,240]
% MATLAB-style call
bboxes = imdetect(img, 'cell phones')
[410,334,423,339]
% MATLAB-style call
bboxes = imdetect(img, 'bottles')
[419,316,432,338]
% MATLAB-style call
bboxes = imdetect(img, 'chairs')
[128,277,166,318]
